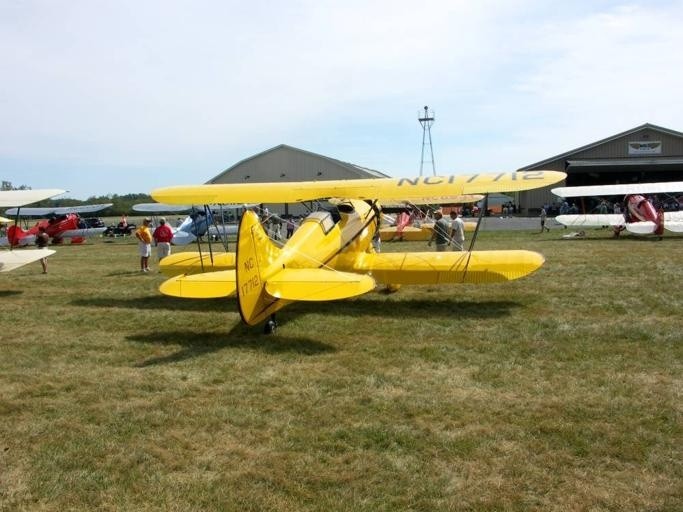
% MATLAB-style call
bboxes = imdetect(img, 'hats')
[159,218,165,222]
[433,210,442,216]
[142,218,150,223]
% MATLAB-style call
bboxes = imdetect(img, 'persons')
[471,204,478,217]
[34,225,48,274]
[647,194,682,210]
[134,216,152,273]
[46,213,57,225]
[369,229,382,253]
[175,217,183,227]
[445,211,465,252]
[500,200,514,218]
[150,217,173,273]
[427,210,450,252]
[538,205,549,233]
[286,217,293,239]
[476,200,482,215]
[542,198,623,215]
[118,213,127,239]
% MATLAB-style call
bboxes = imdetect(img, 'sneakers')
[141,266,153,272]
